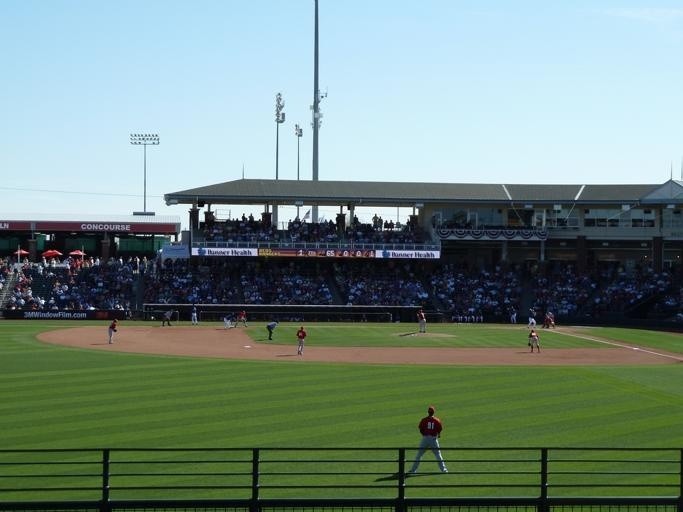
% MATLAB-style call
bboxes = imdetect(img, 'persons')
[415,308,426,334]
[527,329,541,354]
[0,213,681,329]
[409,406,449,474]
[294,326,306,355]
[107,318,117,344]
[265,318,280,341]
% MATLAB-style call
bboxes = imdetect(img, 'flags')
[302,210,310,219]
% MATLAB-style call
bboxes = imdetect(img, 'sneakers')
[408,468,449,474]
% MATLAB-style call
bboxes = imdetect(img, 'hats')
[428,405,435,413]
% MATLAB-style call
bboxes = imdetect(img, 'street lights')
[295,124,302,180]
[275,92,286,179]
[130,133,159,212]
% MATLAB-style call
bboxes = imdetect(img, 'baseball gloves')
[528,343,531,346]
[114,329,117,332]
